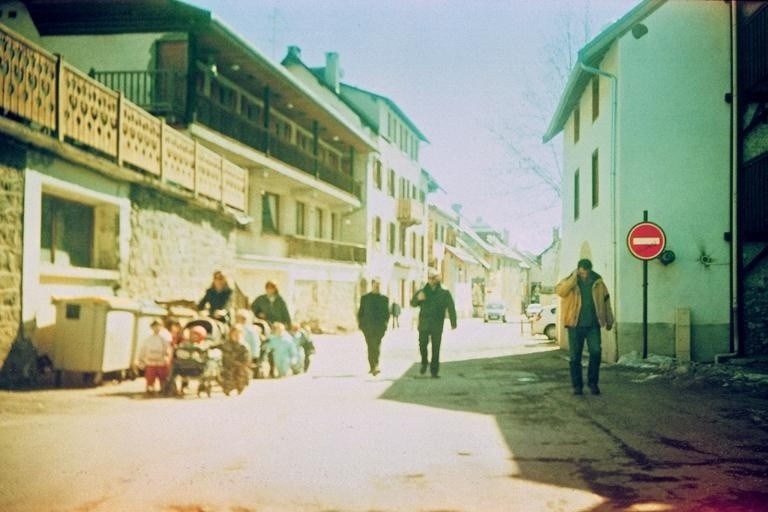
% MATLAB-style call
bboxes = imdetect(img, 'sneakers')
[573,385,600,395]
[420,365,441,379]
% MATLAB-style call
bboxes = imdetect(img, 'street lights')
[580,60,618,361]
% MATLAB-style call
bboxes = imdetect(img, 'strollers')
[163,313,272,399]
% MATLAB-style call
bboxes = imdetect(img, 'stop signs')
[626,222,666,261]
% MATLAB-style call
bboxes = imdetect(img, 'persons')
[250,280,296,331]
[139,305,314,400]
[192,271,235,322]
[554,257,614,397]
[406,266,459,380]
[356,277,391,375]
[388,297,402,328]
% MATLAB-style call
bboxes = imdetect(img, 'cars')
[532,306,557,341]
[525,303,541,317]
[484,301,507,324]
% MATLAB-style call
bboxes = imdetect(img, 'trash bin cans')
[49,296,198,387]
